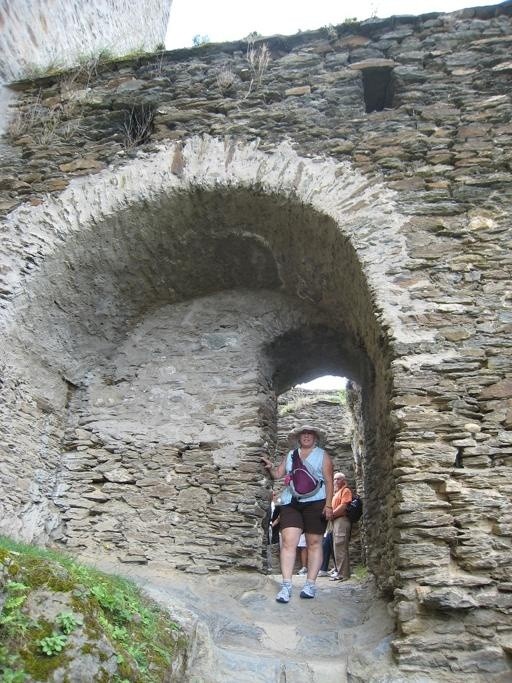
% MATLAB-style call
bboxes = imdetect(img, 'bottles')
[286,425,328,450]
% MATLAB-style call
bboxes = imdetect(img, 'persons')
[269,472,352,581]
[260,424,334,603]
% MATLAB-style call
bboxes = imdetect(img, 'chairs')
[318,568,345,581]
[276,582,292,603]
[297,567,307,575]
[300,581,316,598]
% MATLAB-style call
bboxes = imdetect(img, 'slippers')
[341,487,363,522]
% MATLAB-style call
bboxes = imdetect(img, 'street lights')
[290,450,323,500]
